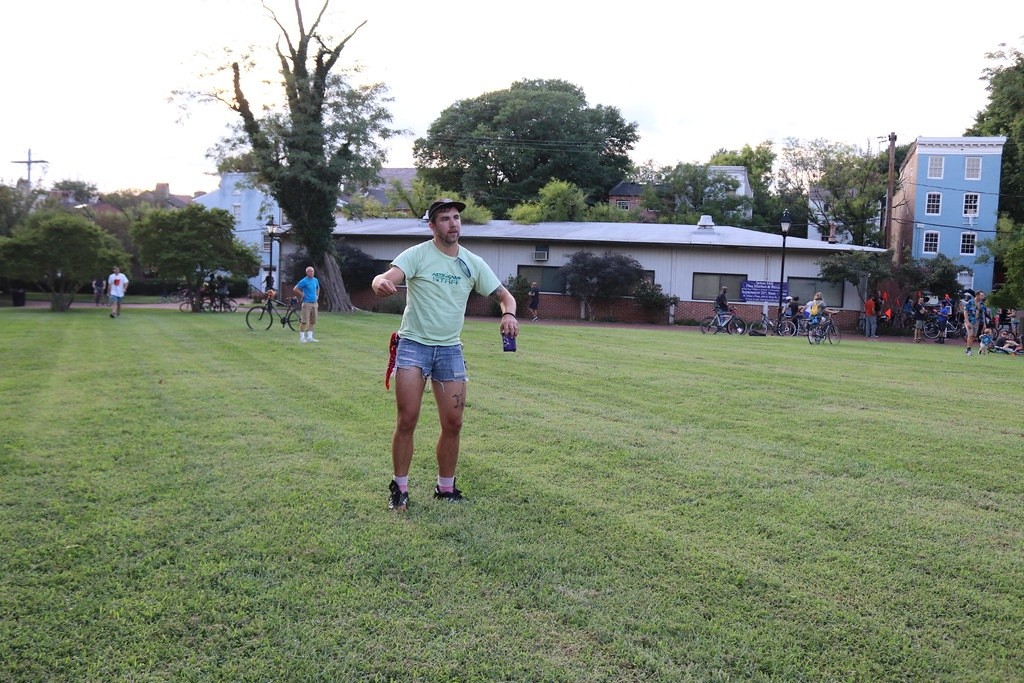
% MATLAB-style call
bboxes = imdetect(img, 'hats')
[429,199,466,220]
[786,296,793,300]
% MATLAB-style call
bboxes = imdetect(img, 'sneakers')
[433,477,471,503]
[387,480,409,514]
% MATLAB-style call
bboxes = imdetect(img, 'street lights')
[779,208,793,326]
[266,215,279,311]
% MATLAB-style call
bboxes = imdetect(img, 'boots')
[934,336,945,344]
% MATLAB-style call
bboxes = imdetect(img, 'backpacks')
[810,300,823,316]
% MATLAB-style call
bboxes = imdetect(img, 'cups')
[502,331,516,352]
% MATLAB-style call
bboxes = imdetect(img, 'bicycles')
[749,312,796,336]
[905,308,1004,341]
[798,313,842,345]
[700,307,747,335]
[246,290,310,333]
[162,286,239,313]
[857,313,889,334]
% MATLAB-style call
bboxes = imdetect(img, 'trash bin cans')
[13,288,26,306]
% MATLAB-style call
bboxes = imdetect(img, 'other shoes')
[300,338,319,343]
[110,313,120,318]
[965,350,972,356]
[865,335,879,338]
[1011,352,1016,356]
[1016,346,1023,350]
[531,316,540,323]
[979,349,985,355]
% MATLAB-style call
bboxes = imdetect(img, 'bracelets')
[502,313,518,319]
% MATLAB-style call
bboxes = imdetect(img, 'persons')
[716,286,1023,353]
[93,265,129,317]
[978,329,993,355]
[528,282,539,322]
[964,291,986,356]
[261,272,274,292]
[293,266,319,343]
[372,198,519,510]
[208,273,227,312]
